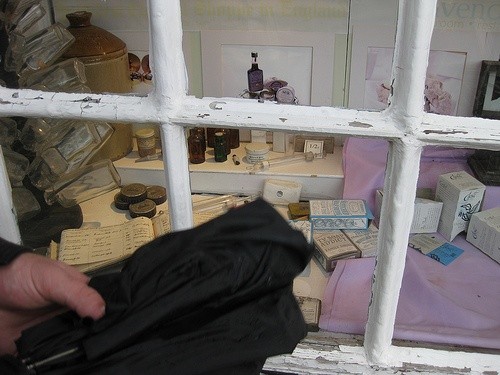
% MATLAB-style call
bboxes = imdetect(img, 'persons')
[0,237,105,359]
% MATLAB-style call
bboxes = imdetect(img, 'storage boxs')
[309,198,376,231]
[287,219,314,244]
[311,230,362,273]
[466,206,500,264]
[341,221,379,258]
[374,188,445,234]
[434,171,488,243]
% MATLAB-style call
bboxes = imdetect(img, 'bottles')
[246,142,271,164]
[61,10,133,165]
[214,132,227,162]
[187,127,206,164]
[135,128,155,159]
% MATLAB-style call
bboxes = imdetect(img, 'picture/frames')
[111,30,194,138]
[200,30,336,143]
[347,24,486,117]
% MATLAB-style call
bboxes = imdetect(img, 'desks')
[44,141,500,375]
